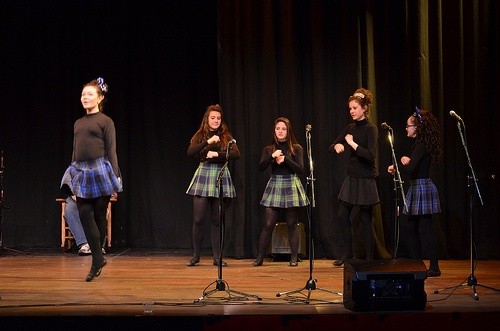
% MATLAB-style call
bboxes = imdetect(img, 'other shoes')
[78,244,91,256]
[253,259,263,265]
[427,270,440,277]
[86,258,106,282]
[186,257,200,265]
[333,256,347,265]
[290,260,298,266]
[213,260,227,267]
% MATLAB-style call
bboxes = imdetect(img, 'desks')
[56,197,117,254]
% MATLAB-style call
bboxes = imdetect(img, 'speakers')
[344,259,428,312]
[271,221,306,259]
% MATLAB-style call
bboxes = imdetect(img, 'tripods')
[193,145,263,303]
[434,119,500,301]
[276,132,343,305]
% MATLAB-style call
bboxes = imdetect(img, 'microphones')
[306,124,312,132]
[381,122,391,130]
[450,111,463,121]
[228,139,236,146]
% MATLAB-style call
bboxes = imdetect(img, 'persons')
[253,118,310,266]
[185,104,240,266]
[60,165,107,255]
[387,109,443,277]
[329,88,380,266]
[71,78,124,281]
